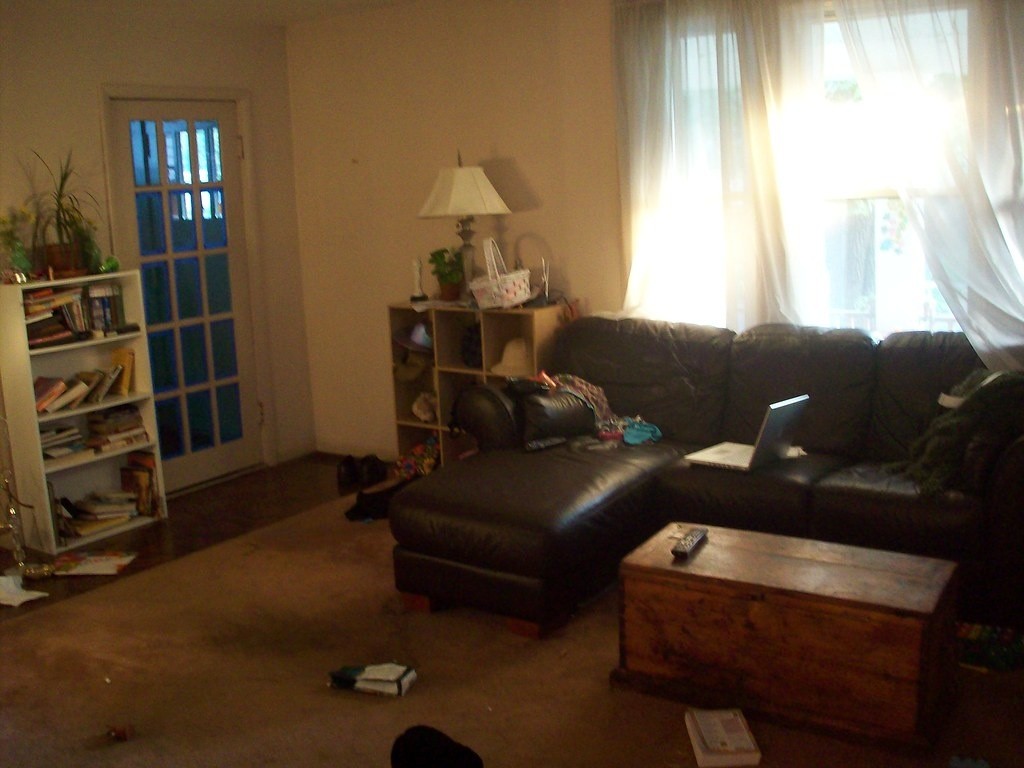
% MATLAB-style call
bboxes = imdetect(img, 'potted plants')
[427,247,463,301]
[21,147,107,273]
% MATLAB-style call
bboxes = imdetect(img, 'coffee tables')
[610,522,964,757]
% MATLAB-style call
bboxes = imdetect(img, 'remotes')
[526,437,566,451]
[671,528,709,558]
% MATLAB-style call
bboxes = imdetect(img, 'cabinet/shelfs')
[2,269,169,557]
[386,290,582,468]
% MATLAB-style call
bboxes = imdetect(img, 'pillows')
[524,392,595,441]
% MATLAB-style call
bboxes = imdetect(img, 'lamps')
[416,149,513,306]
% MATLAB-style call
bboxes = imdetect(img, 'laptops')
[684,393,810,470]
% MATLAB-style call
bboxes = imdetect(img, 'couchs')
[388,315,1023,642]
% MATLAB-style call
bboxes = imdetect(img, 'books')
[40,404,148,468]
[47,452,160,545]
[35,348,134,412]
[685,708,761,768]
[23,282,126,350]
